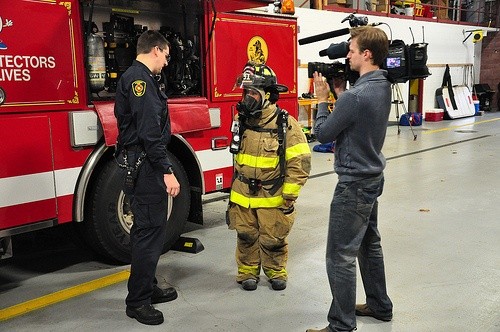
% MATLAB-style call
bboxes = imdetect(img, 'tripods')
[392,83,417,141]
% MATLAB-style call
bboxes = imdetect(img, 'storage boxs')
[425,108,445,122]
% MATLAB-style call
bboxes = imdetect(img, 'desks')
[298,99,337,135]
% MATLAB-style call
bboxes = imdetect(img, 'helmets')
[238,63,288,93]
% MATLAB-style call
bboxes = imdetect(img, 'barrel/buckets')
[473,100,480,115]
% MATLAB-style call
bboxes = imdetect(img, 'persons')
[226,62,311,290]
[114,30,180,325]
[306,27,393,332]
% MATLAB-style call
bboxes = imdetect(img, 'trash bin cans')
[473,84,495,111]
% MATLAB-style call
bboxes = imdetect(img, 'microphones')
[298,28,351,45]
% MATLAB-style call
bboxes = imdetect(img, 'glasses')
[154,45,171,63]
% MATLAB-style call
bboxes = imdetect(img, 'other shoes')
[126,305,164,325]
[242,278,257,291]
[307,327,358,332]
[272,279,286,290]
[356,304,392,321]
[151,287,177,304]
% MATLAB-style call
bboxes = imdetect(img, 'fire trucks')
[0,0,301,264]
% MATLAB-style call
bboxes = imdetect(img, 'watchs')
[163,166,174,174]
